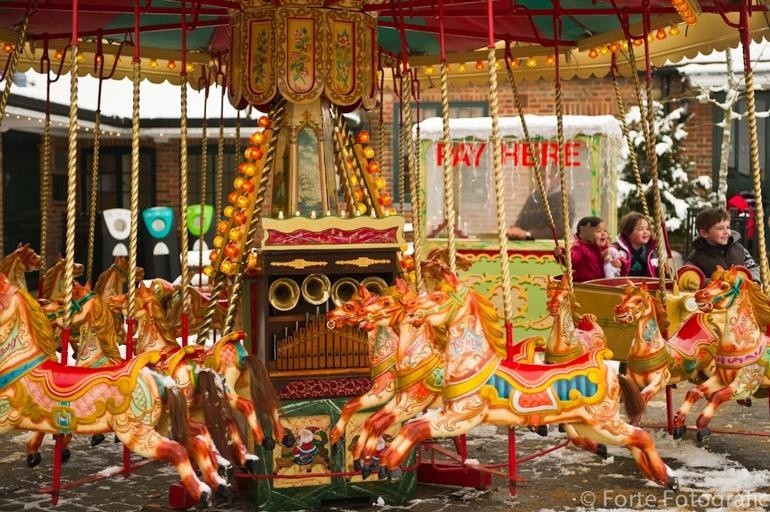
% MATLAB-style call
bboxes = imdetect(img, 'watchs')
[526,230,532,240]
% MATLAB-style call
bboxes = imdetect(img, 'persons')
[727,193,755,240]
[682,207,761,292]
[506,161,576,241]
[613,211,671,278]
[553,216,630,282]
